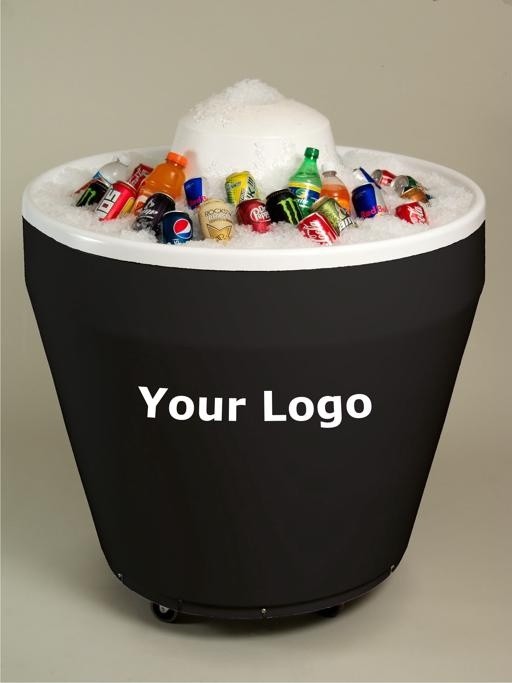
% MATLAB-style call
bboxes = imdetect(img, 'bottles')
[132,153,184,221]
[289,147,351,217]
[94,155,127,186]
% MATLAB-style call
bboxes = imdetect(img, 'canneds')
[353,167,432,205]
[310,194,360,238]
[266,188,303,228]
[396,201,429,227]
[129,162,154,191]
[234,199,273,234]
[226,170,261,205]
[163,210,193,244]
[351,182,390,222]
[197,198,235,242]
[76,180,109,209]
[297,210,340,247]
[182,177,212,210]
[96,178,137,224]
